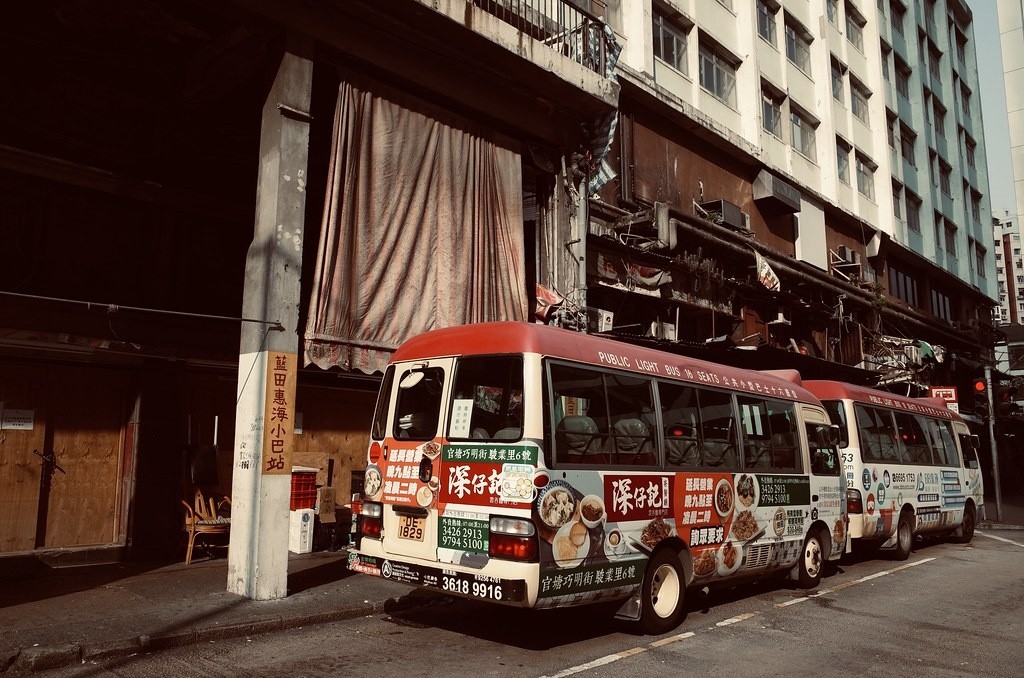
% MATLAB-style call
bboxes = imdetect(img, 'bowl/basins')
[537,479,576,528]
[533,467,551,489]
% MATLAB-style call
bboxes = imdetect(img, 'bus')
[347,320,851,635]
[794,378,984,561]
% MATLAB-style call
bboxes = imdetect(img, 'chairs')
[177,488,232,565]
[861,428,940,465]
[471,411,798,472]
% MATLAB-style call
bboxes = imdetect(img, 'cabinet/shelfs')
[0,356,142,577]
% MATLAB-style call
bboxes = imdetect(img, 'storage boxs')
[289,466,320,511]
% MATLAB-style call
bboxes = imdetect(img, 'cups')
[608,527,627,547]
[579,494,607,529]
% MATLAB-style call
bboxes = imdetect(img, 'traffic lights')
[972,377,989,419]
[994,385,1019,419]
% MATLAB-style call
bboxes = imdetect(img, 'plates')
[733,474,760,513]
[692,553,720,577]
[629,519,676,552]
[730,513,768,547]
[717,538,743,576]
[604,534,626,561]
[714,478,734,516]
[552,521,591,568]
